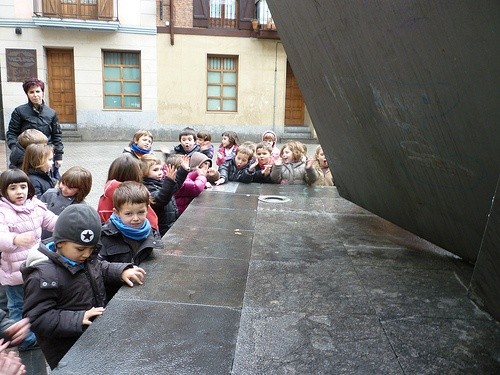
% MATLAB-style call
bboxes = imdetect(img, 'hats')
[53,204,101,249]
[190,151,212,169]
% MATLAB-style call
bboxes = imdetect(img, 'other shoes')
[18,339,41,351]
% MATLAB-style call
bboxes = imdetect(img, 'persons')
[135,153,191,238]
[9,128,48,171]
[122,129,154,161]
[5,77,64,182]
[0,309,33,375]
[160,127,336,216]
[21,143,63,199]
[98,155,161,239]
[0,169,60,349]
[98,181,164,307]
[41,167,92,239]
[20,204,146,371]
